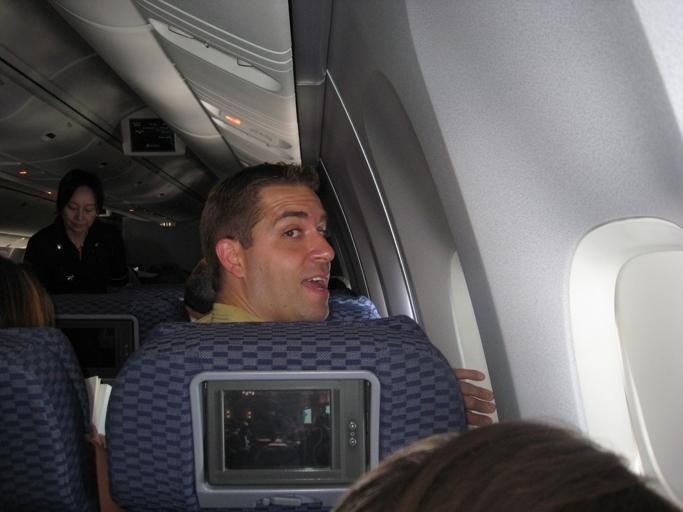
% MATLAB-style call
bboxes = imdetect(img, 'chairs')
[47,279,382,323]
[3,327,91,511]
[105,315,471,509]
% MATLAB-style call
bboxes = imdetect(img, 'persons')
[0,254,126,512]
[325,418,679,511]
[186,158,338,324]
[22,166,131,296]
[184,256,216,316]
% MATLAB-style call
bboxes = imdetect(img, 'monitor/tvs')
[207,381,367,486]
[120,111,185,157]
[56,320,133,378]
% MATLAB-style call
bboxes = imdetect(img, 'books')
[82,375,113,440]
